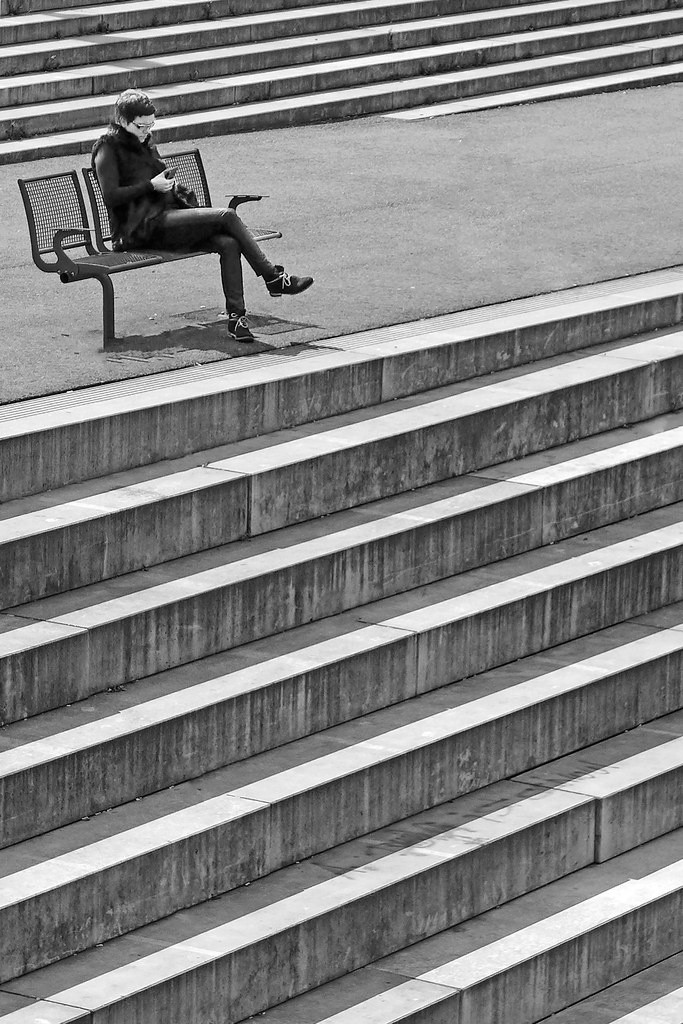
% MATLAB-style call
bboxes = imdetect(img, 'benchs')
[17,148,282,348]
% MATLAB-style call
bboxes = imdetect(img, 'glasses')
[132,121,154,129]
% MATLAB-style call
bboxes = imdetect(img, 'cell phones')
[165,167,179,179]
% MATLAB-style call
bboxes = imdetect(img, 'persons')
[90,89,313,340]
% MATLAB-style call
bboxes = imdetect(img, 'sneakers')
[266,265,313,297]
[227,309,254,342]
